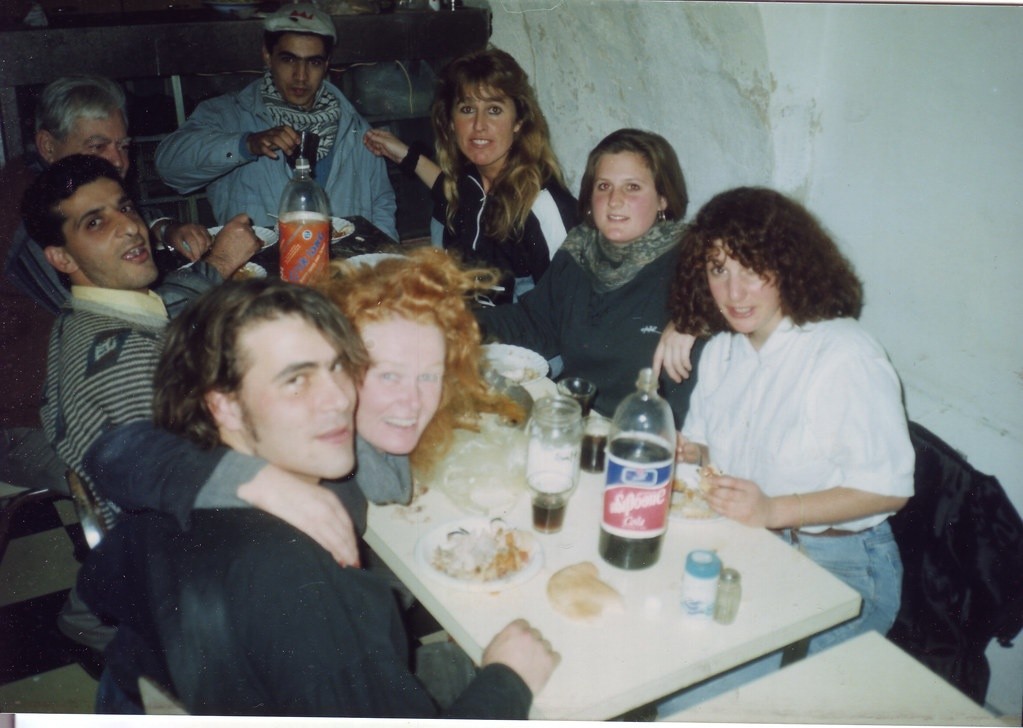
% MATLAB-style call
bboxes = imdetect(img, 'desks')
[140,216,1007,728]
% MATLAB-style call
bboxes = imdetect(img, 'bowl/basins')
[202,0,265,19]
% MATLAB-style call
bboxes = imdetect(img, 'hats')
[262,0,337,54]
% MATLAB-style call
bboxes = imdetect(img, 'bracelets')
[398,140,424,176]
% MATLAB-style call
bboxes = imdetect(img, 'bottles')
[597,368,677,570]
[682,549,743,627]
[278,158,331,288]
[520,395,583,497]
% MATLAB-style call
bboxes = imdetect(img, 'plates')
[177,261,268,280]
[671,462,705,507]
[274,216,355,244]
[205,226,277,255]
[478,344,549,386]
[342,252,412,272]
[414,517,545,594]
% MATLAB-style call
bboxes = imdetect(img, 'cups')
[581,416,611,473]
[284,131,319,177]
[526,470,576,535]
[556,377,598,419]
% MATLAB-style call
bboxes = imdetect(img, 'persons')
[0,1,917,719]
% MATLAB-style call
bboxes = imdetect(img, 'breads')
[546,560,624,622]
[696,463,722,497]
